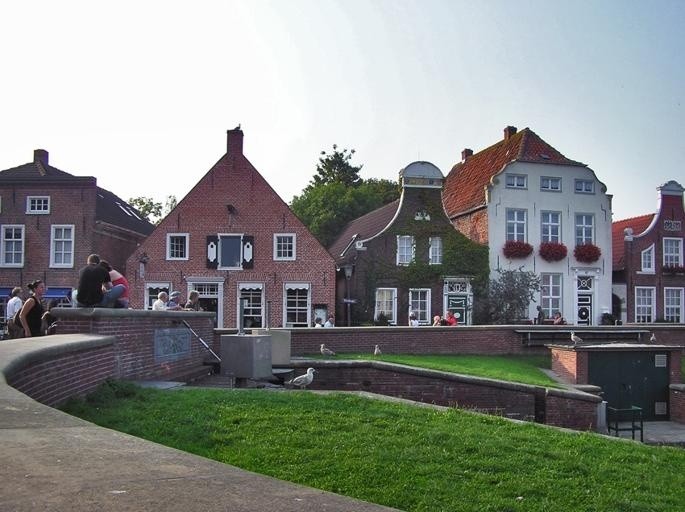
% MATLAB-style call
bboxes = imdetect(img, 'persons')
[184,289,204,310]
[409,312,420,326]
[323,314,336,328]
[313,317,323,328]
[552,312,567,325]
[152,292,181,310]
[432,316,442,326]
[98,260,130,309]
[536,305,545,325]
[166,291,196,311]
[3,278,60,337]
[443,309,458,326]
[71,254,126,308]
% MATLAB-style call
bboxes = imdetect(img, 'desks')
[607,404,645,442]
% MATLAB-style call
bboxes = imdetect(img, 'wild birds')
[283,367,319,390]
[649,332,657,344]
[374,344,382,356]
[319,342,338,358]
[570,330,583,346]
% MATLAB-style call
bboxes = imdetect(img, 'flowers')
[503,238,602,263]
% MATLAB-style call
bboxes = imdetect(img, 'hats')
[171,291,181,296]
[410,312,415,318]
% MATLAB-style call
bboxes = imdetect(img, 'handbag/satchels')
[13,307,29,327]
